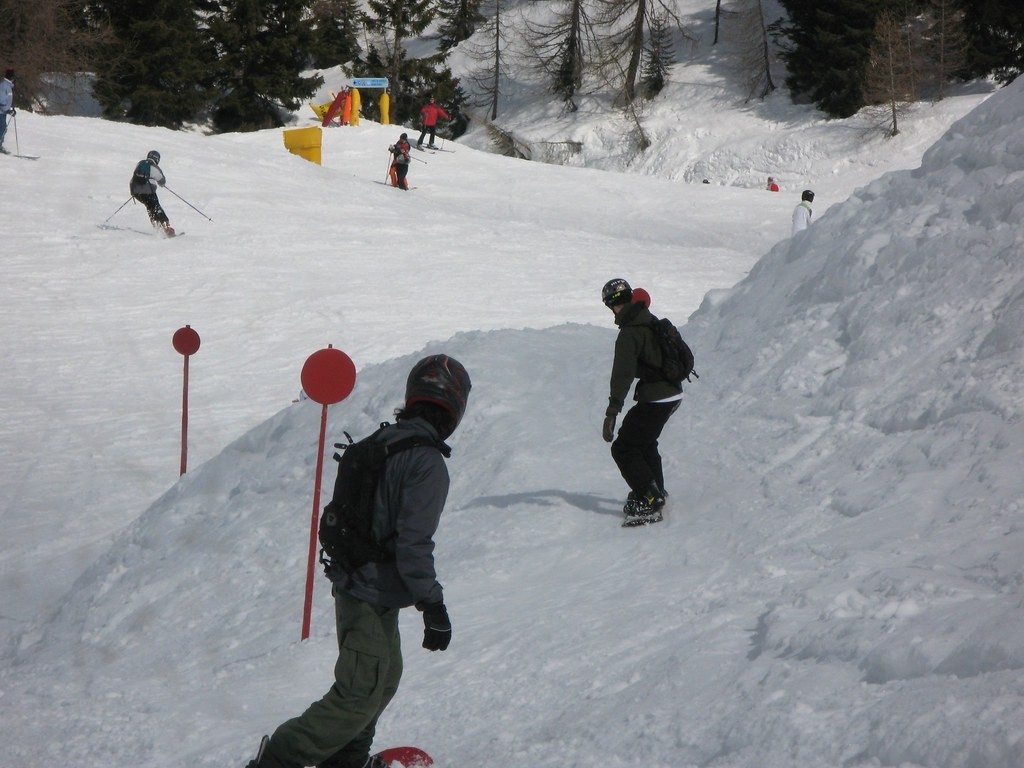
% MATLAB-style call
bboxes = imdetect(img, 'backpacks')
[132,161,151,188]
[316,422,452,592]
[618,316,700,385]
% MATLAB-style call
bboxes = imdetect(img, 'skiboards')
[410,144,457,155]
[372,180,418,192]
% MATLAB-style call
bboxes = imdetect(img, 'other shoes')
[162,220,174,237]
[0,147,10,154]
[622,483,669,526]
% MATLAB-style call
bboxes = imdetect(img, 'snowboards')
[371,748,434,767]
[162,231,186,240]
[621,490,664,530]
[11,154,41,160]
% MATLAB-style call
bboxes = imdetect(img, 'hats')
[400,133,408,140]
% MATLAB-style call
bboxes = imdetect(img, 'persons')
[0,68,17,155]
[129,150,175,235]
[766,176,779,191]
[388,133,411,191]
[792,190,815,236]
[417,95,448,151]
[602,278,684,518]
[243,354,472,768]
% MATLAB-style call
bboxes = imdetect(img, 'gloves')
[601,397,621,442]
[8,110,17,116]
[416,600,452,652]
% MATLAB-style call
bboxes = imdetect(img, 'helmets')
[147,151,160,166]
[5,67,15,76]
[405,355,471,443]
[601,279,633,307]
[802,189,814,203]
